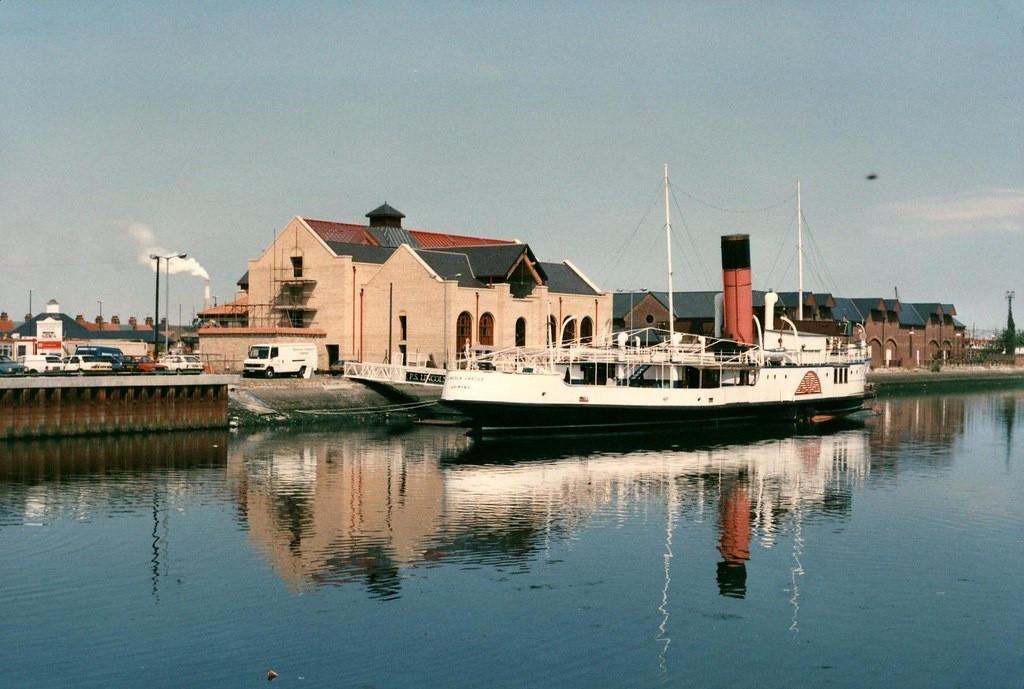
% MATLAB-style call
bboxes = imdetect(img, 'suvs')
[15,355,66,373]
[76,346,133,371]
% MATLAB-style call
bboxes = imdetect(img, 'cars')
[0,355,22,374]
[160,356,205,375]
[123,354,172,372]
[61,355,113,373]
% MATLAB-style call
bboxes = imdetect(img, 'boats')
[338,357,458,414]
[433,160,874,421]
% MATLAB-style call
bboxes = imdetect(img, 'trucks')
[242,342,319,380]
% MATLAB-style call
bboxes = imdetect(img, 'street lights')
[150,255,160,361]
[97,300,102,332]
[164,255,188,356]
[29,288,36,336]
